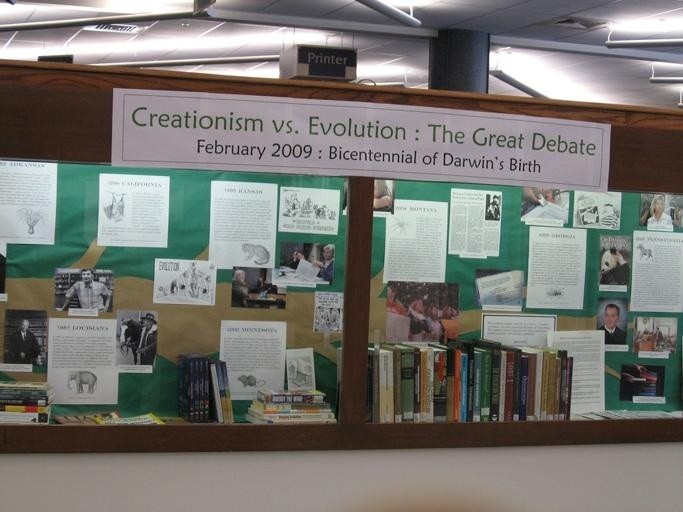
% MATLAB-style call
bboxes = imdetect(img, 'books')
[52,412,120,425]
[0,382,56,424]
[94,413,165,425]
[337,337,574,422]
[210,361,235,423]
[244,390,337,425]
[178,356,209,422]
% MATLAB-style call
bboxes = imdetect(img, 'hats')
[139,313,157,326]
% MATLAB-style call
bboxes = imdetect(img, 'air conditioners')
[82,24,137,33]
[544,15,609,33]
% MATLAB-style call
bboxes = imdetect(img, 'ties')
[22,333,26,341]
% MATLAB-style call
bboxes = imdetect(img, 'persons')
[55,269,110,312]
[386,285,458,341]
[136,313,158,365]
[487,196,500,220]
[598,304,626,345]
[373,179,393,211]
[286,249,304,270]
[7,318,41,362]
[622,364,648,384]
[521,188,553,214]
[232,270,250,307]
[633,328,677,352]
[640,195,673,232]
[316,246,334,282]
